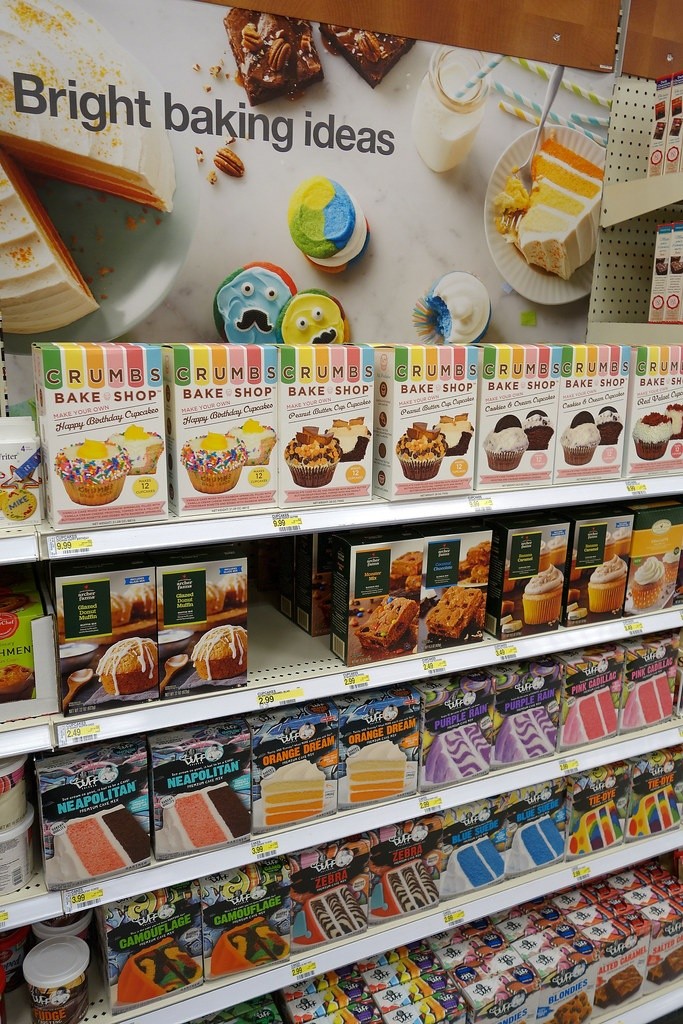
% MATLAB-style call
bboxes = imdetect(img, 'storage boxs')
[294,532,333,638]
[0,590,44,705]
[0,415,45,529]
[472,345,563,492]
[417,527,491,653]
[559,511,634,629]
[622,346,683,478]
[162,344,277,517]
[273,346,374,508]
[278,537,297,623]
[32,629,683,1024]
[36,345,169,528]
[483,520,571,640]
[329,529,424,669]
[372,346,478,501]
[149,546,248,702]
[621,500,683,616]
[648,70,683,325]
[49,558,160,718]
[552,344,631,485]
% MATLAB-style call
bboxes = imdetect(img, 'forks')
[493,64,566,231]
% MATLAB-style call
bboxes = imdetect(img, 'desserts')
[0,410,683,1024]
[224,7,419,106]
[212,176,491,345]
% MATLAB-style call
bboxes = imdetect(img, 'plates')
[3,51,197,355]
[483,125,607,306]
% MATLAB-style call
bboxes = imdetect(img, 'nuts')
[214,148,244,178]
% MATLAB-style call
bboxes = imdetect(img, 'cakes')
[493,138,605,280]
[0,0,175,339]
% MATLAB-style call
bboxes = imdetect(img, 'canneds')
[0,926,30,994]
[22,935,90,1023]
[0,754,28,834]
[30,908,93,944]
[0,802,35,896]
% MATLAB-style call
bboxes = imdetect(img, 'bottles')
[410,43,490,172]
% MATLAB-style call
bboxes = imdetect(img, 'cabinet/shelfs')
[0,475,683,1024]
